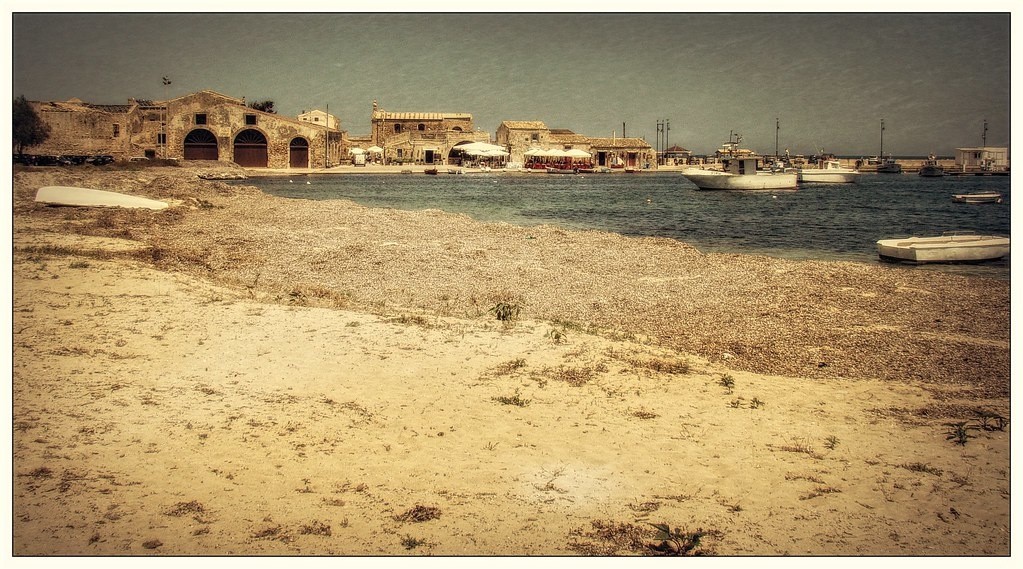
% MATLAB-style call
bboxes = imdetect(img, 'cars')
[14,151,113,166]
[127,156,148,163]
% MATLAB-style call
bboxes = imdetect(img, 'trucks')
[352,153,366,166]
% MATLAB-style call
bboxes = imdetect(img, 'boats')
[447,168,463,174]
[975,156,1010,176]
[681,129,797,190]
[951,189,1002,204]
[783,148,861,183]
[579,167,597,174]
[877,230,1011,266]
[876,152,902,172]
[424,167,438,174]
[546,167,576,174]
[917,150,943,176]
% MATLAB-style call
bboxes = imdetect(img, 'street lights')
[161,76,172,158]
